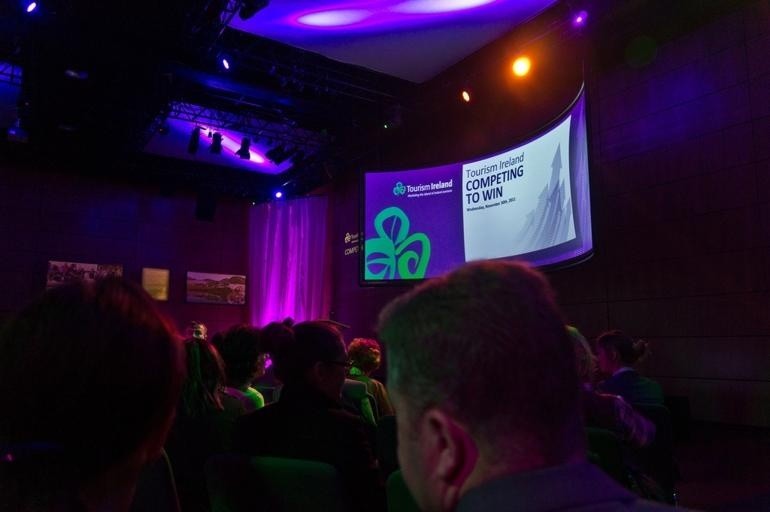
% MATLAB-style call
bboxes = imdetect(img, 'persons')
[377,260,682,510]
[1,256,397,511]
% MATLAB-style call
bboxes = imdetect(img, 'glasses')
[326,356,355,376]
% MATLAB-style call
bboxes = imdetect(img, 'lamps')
[187,126,298,165]
